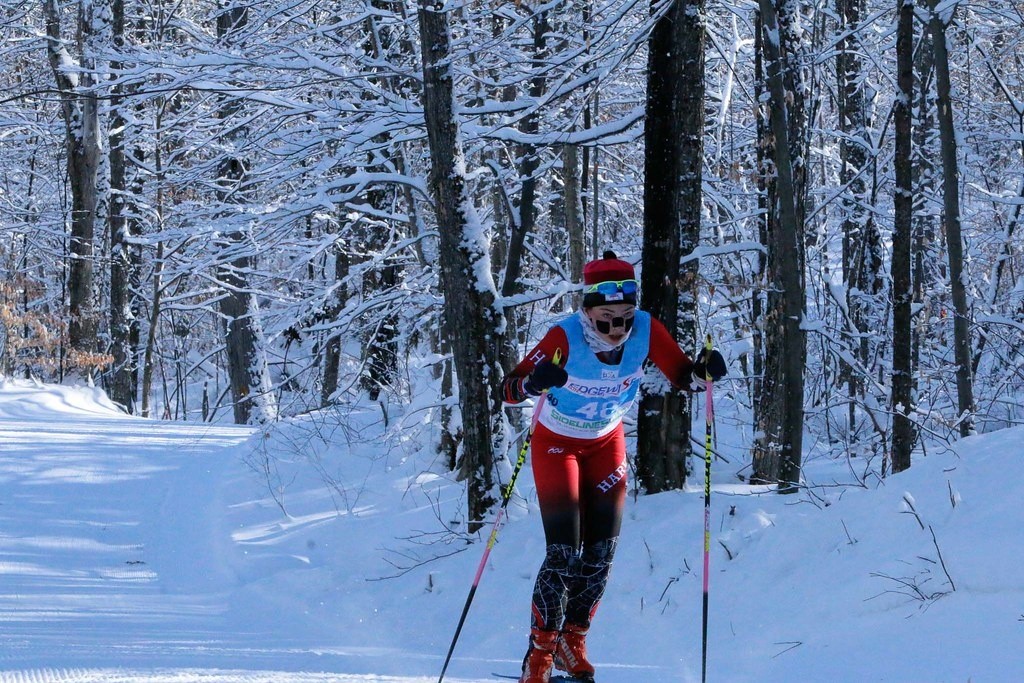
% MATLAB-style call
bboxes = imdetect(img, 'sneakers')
[519,628,560,683]
[554,618,595,676]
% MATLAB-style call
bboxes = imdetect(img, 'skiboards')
[490,672,595,683]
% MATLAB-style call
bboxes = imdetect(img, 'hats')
[583,251,636,307]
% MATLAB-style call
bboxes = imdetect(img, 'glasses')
[588,279,638,294]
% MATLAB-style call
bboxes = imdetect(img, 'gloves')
[522,355,568,397]
[692,346,726,383]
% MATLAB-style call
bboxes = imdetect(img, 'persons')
[501,250,728,683]
